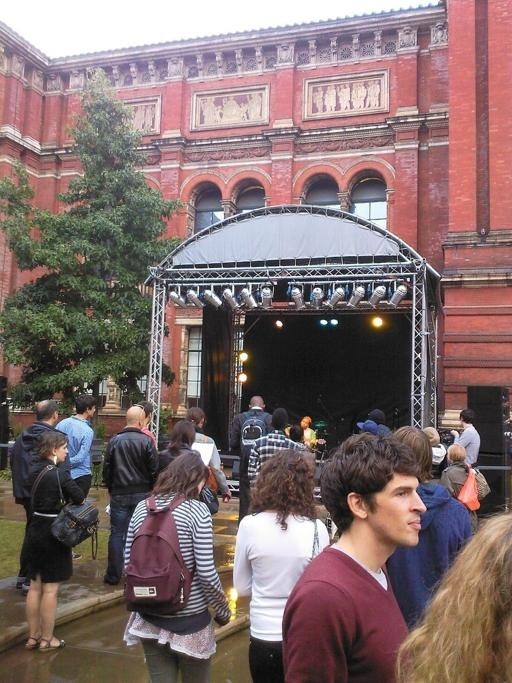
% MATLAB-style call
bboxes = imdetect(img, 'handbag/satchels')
[50,503,99,547]
[196,462,224,515]
[457,465,491,512]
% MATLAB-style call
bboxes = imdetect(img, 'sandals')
[25,635,66,652]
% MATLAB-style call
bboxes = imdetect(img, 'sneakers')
[16,582,29,595]
[104,573,120,585]
[72,551,83,560]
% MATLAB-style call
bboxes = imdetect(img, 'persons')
[233,449,330,683]
[55,396,96,561]
[10,400,70,596]
[103,401,232,584]
[230,396,317,528]
[392,506,511,682]
[355,408,480,630]
[18,429,84,652]
[281,432,427,682]
[122,453,232,683]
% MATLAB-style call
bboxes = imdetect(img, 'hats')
[422,427,440,446]
[356,409,391,436]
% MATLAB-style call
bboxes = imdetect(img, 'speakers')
[471,454,509,518]
[0,374,9,471]
[467,385,509,454]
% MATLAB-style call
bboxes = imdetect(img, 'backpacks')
[239,412,268,457]
[125,493,196,613]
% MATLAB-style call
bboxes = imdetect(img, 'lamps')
[167,285,408,315]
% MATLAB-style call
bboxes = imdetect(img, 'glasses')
[131,404,144,421]
